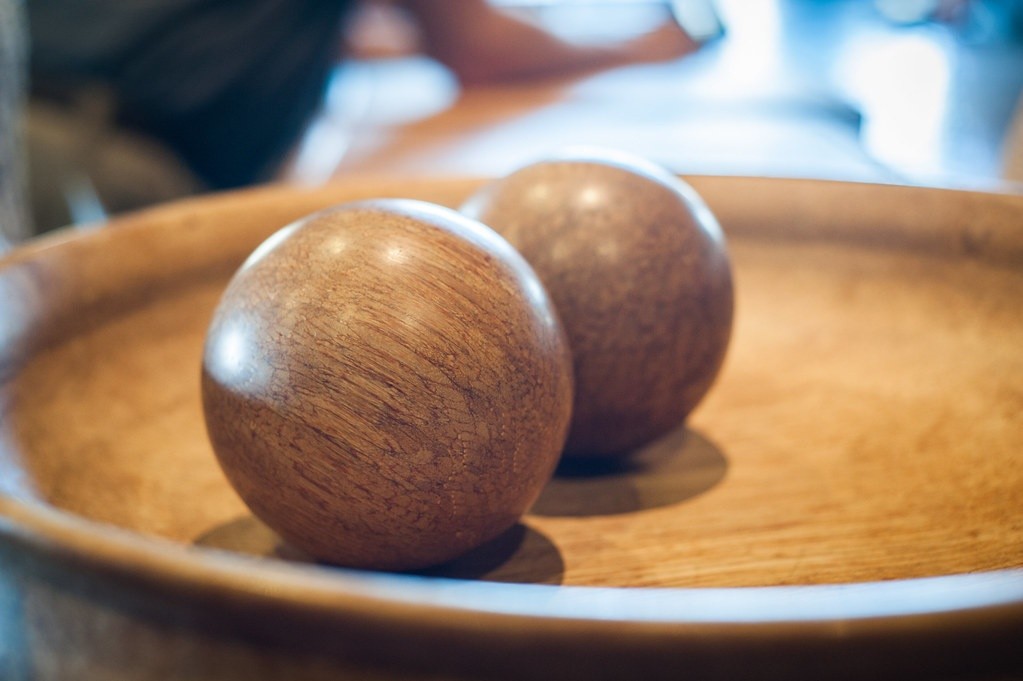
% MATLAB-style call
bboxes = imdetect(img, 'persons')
[0,0,706,239]
